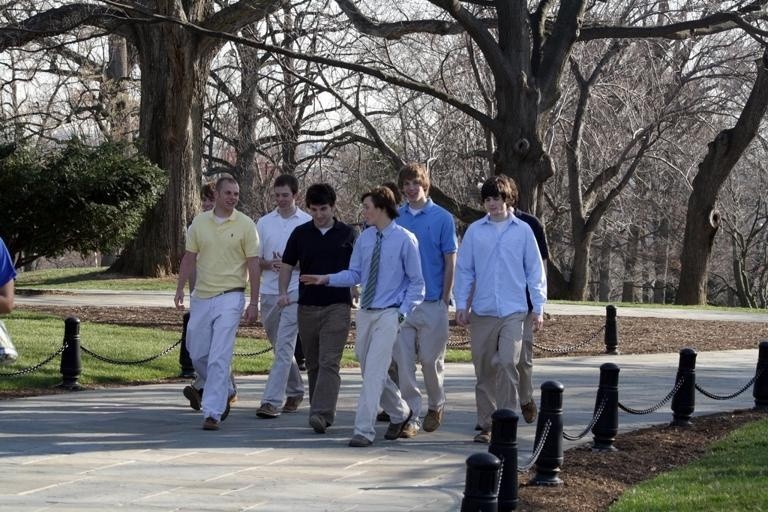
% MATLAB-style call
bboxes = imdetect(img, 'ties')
[361,232,383,309]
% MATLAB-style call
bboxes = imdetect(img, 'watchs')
[398,312,404,323]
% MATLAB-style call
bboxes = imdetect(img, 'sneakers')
[256,402,280,418]
[521,399,537,423]
[309,414,326,434]
[282,395,303,414]
[474,432,491,442]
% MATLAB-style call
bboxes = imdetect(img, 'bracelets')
[249,301,258,307]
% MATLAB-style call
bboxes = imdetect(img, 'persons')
[255,173,315,418]
[376,178,407,421]
[383,161,458,440]
[495,175,539,424]
[0,236,21,370]
[184,176,263,430]
[276,182,360,432]
[299,187,428,447]
[452,174,546,444]
[175,180,240,409]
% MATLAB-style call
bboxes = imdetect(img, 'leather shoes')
[349,436,371,446]
[378,410,441,440]
[181,385,237,430]
